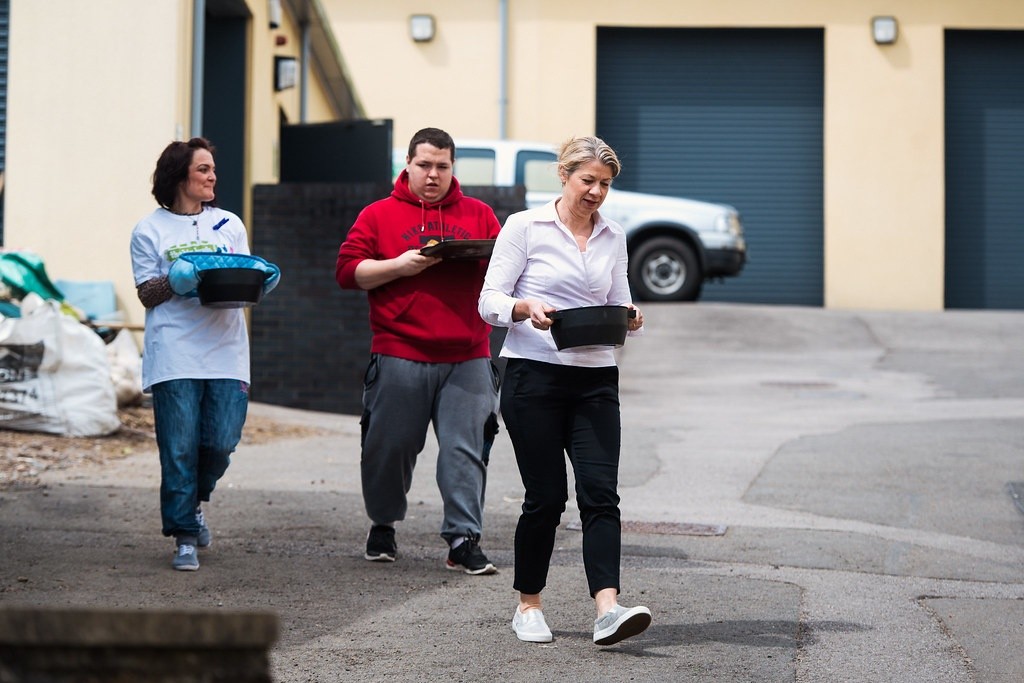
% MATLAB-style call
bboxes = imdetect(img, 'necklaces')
[188,211,200,225]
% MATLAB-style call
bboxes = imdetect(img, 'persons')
[476,136,652,646]
[129,138,281,570]
[337,127,501,574]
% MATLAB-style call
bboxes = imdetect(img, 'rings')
[634,324,635,327]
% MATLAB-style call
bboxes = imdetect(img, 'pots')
[195,269,274,309]
[546,306,636,353]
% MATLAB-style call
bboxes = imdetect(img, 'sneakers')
[364,524,398,562]
[446,528,497,575]
[172,544,200,571]
[511,603,553,643]
[194,506,212,549]
[593,602,652,646]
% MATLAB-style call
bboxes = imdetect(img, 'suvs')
[394,143,747,303]
